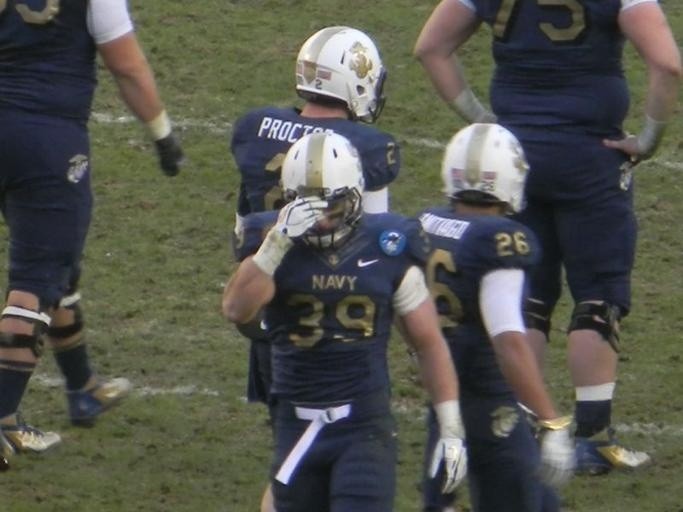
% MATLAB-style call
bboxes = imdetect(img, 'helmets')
[296,25,388,125]
[442,123,528,214]
[281,131,364,252]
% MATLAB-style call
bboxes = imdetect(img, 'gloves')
[429,439,468,494]
[156,134,182,176]
[540,430,575,486]
[277,195,327,238]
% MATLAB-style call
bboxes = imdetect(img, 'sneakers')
[65,377,129,427]
[575,438,651,475]
[0,424,61,458]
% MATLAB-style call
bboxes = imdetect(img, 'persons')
[0,2,185,454]
[220,132,470,510]
[411,123,573,510]
[231,24,400,409]
[412,2,683,472]
